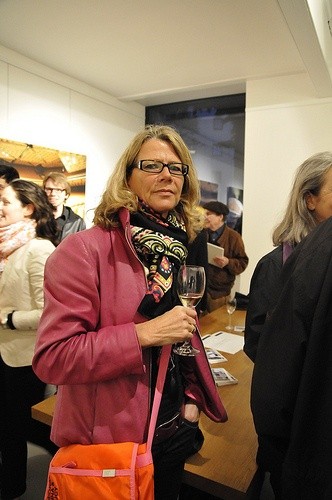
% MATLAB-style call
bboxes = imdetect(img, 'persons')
[0,164,87,500]
[32,126,229,500]
[202,151,332,500]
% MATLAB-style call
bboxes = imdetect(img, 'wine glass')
[173,265,206,356]
[225,297,237,329]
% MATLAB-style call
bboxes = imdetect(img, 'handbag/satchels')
[44,441,154,500]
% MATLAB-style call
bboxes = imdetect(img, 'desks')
[32,305,266,500]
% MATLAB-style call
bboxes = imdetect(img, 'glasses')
[131,159,189,175]
[44,187,66,194]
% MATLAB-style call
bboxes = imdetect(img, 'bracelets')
[0,311,11,330]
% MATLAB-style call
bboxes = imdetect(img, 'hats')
[203,202,229,215]
